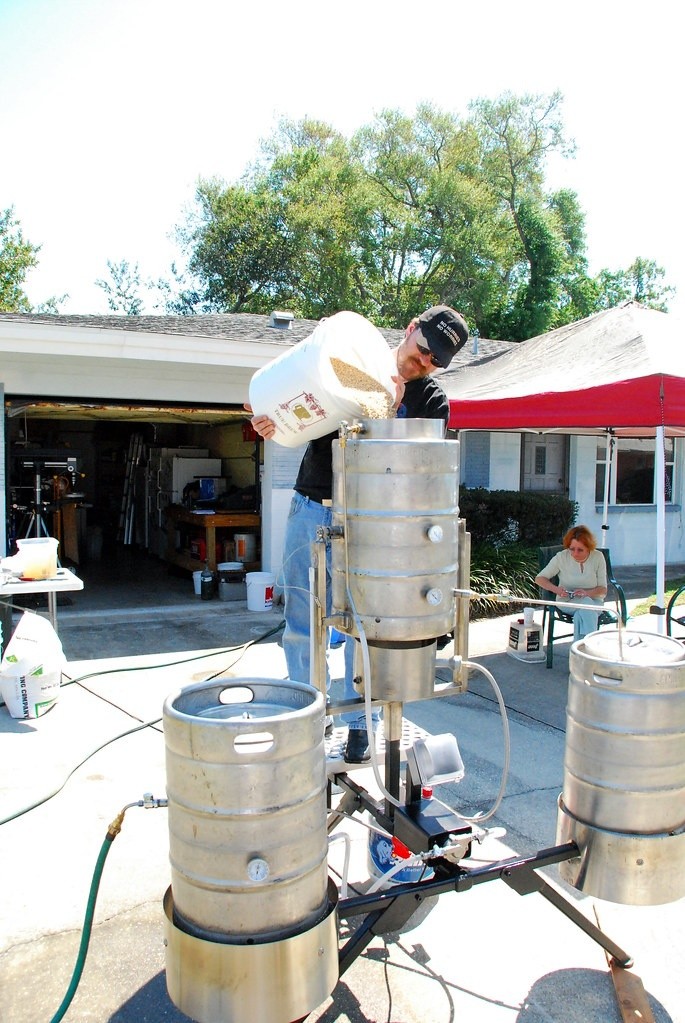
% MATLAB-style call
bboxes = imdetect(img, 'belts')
[297,489,333,507]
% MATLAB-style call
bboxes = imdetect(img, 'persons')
[535,525,607,642]
[243,305,469,764]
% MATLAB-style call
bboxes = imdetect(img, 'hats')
[419,305,469,369]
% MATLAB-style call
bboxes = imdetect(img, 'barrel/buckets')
[506,623,546,663]
[191,541,200,559]
[369,796,433,884]
[249,311,397,448]
[200,539,221,563]
[193,571,214,595]
[16,536,59,580]
[234,533,256,562]
[222,540,234,562]
[246,572,274,612]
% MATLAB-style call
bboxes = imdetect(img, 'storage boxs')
[16,538,59,579]
[508,622,548,663]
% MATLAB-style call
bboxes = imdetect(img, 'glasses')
[570,546,587,552]
[416,324,443,368]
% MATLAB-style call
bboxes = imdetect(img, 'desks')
[170,503,259,588]
[0,567,83,684]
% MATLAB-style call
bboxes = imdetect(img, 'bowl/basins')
[218,570,246,583]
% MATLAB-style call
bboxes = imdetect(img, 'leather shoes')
[325,722,334,734]
[343,728,377,764]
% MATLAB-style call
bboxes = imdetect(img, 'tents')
[429,299,685,639]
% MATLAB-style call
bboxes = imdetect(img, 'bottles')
[201,559,213,600]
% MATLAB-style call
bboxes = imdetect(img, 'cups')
[524,608,534,626]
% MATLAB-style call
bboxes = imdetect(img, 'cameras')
[565,590,575,599]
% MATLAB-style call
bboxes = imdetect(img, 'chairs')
[540,543,627,668]
